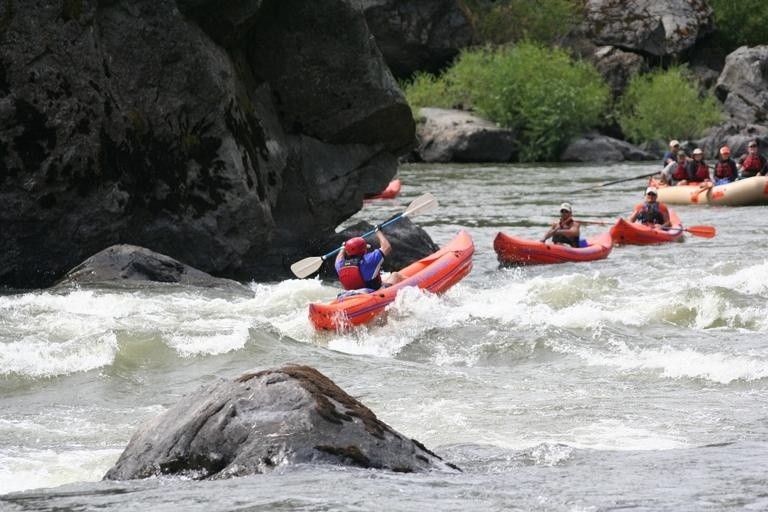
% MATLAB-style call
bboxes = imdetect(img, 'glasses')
[648,194,656,196]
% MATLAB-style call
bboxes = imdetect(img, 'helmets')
[691,148,704,159]
[344,237,368,256]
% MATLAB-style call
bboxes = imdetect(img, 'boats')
[492,232,614,265]
[309,229,473,335]
[610,208,684,243]
[645,177,768,206]
[368,179,399,201]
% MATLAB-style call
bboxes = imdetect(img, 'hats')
[720,146,730,155]
[646,187,657,195]
[669,140,680,148]
[677,150,685,156]
[748,141,758,146]
[560,202,572,211]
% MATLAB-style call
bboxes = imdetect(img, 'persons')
[630,186,672,231]
[715,146,738,185]
[335,224,408,292]
[735,141,768,180]
[658,140,710,186]
[545,203,579,248]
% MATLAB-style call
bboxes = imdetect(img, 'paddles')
[290,192,438,279]
[574,220,716,238]
